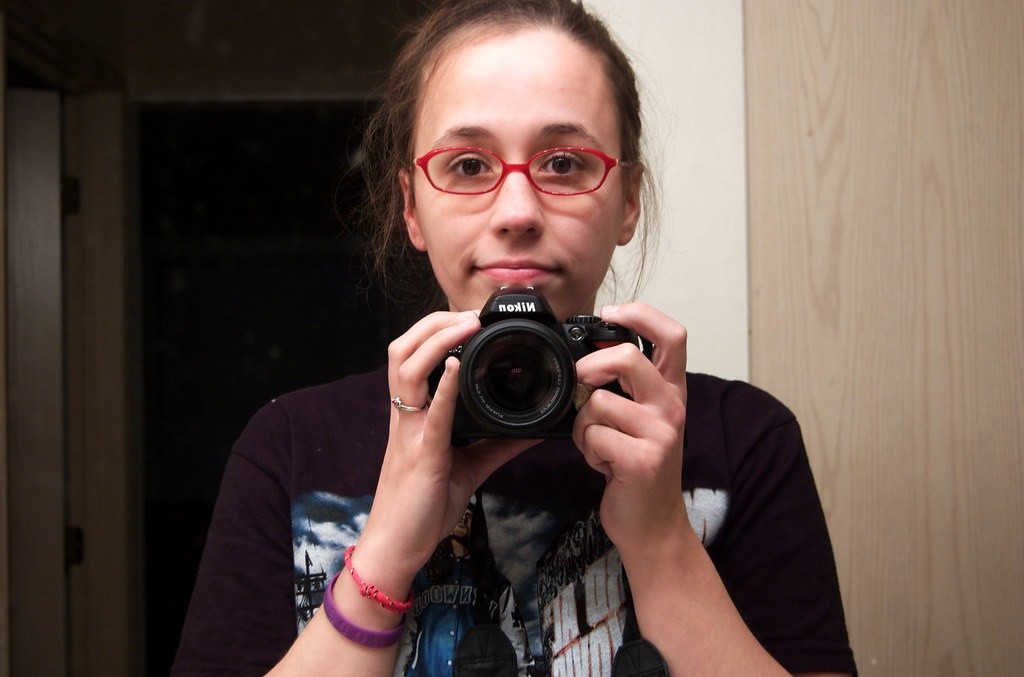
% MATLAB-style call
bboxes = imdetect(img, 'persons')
[164,1,859,676]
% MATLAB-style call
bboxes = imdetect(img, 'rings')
[389,398,422,412]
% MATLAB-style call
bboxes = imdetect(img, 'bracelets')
[343,548,414,613]
[323,572,406,647]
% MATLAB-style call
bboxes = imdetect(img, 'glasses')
[407,146,632,197]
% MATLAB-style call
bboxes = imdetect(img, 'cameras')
[429,285,636,440]
